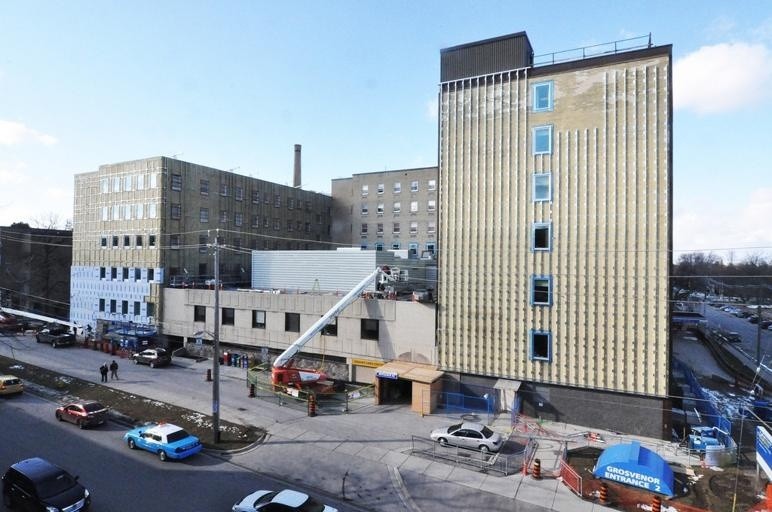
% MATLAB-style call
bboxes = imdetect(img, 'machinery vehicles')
[272,266,398,401]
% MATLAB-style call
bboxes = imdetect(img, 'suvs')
[2,457,92,512]
[133,347,172,369]
[56,399,109,429]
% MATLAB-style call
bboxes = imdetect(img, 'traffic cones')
[520,462,530,477]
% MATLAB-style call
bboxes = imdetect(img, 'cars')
[0,313,27,333]
[431,421,502,453]
[232,489,339,511]
[0,375,24,396]
[707,301,771,344]
[689,425,720,455]
[122,420,203,461]
[36,327,75,348]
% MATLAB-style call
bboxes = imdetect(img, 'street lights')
[193,329,221,444]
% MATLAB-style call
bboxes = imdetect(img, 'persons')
[99,362,108,383]
[376,282,394,299]
[109,360,119,380]
